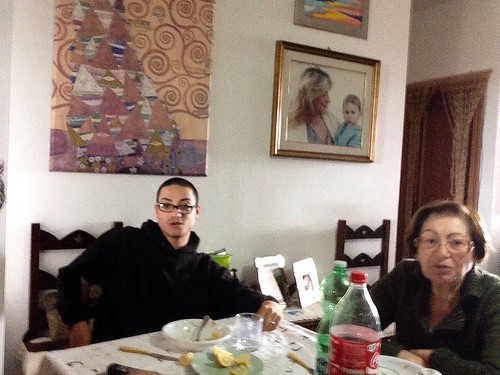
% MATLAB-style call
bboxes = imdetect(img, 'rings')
[270,320,274,324]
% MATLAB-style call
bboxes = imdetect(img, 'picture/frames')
[254,255,292,312]
[293,0,370,39]
[49,0,216,176]
[270,39,382,163]
[292,257,322,308]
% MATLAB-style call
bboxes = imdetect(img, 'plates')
[192,350,263,375]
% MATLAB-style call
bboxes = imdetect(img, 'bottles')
[315,261,350,375]
[327,271,381,375]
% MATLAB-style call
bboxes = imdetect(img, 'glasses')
[156,202,197,213]
[413,236,474,256]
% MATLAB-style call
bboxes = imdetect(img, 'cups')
[235,313,263,351]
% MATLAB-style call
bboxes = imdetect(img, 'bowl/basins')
[162,319,234,351]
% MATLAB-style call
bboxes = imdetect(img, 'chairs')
[335,219,391,290]
[21,221,125,375]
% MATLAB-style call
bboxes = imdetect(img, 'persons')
[354,199,500,375]
[286,66,341,146]
[331,94,363,148]
[56,177,283,349]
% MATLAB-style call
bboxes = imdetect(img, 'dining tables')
[37,312,442,375]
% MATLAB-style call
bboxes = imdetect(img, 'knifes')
[288,353,315,375]
[119,347,178,361]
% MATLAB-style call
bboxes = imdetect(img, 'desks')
[278,296,336,334]
